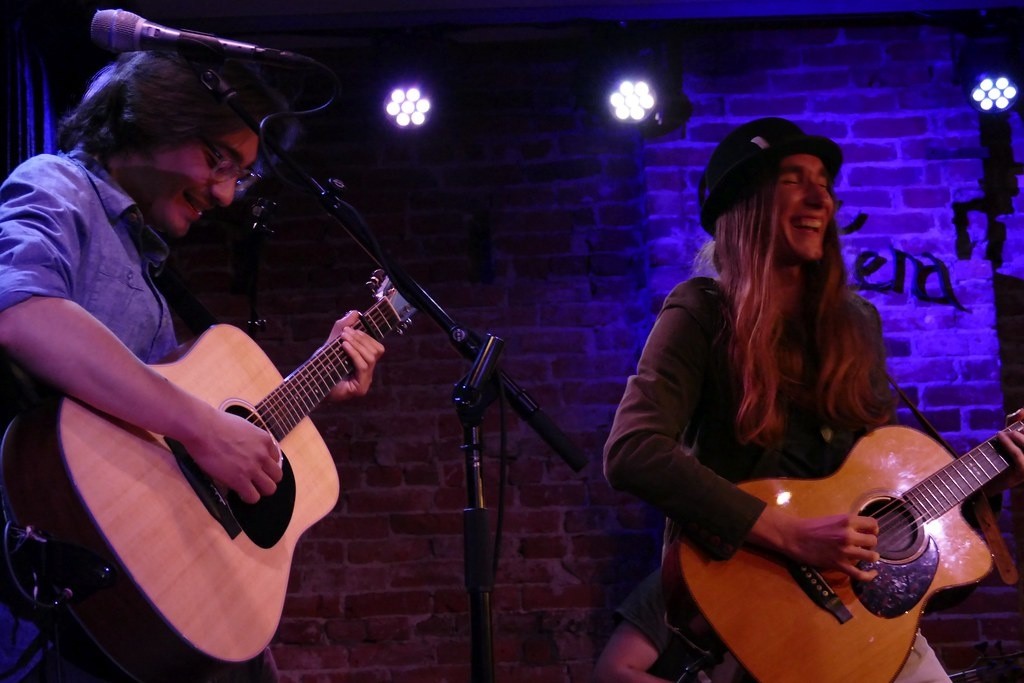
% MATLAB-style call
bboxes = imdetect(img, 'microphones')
[92,8,309,65]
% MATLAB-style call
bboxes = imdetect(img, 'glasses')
[197,138,258,192]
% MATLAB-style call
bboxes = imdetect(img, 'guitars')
[1,266,420,683]
[661,416,1023,683]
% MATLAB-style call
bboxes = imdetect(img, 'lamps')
[598,67,695,139]
[961,38,1022,119]
[380,70,454,140]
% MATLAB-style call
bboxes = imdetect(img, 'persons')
[0,44,387,683]
[597,120,1024,681]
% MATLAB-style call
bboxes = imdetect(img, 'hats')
[701,116,843,240]
